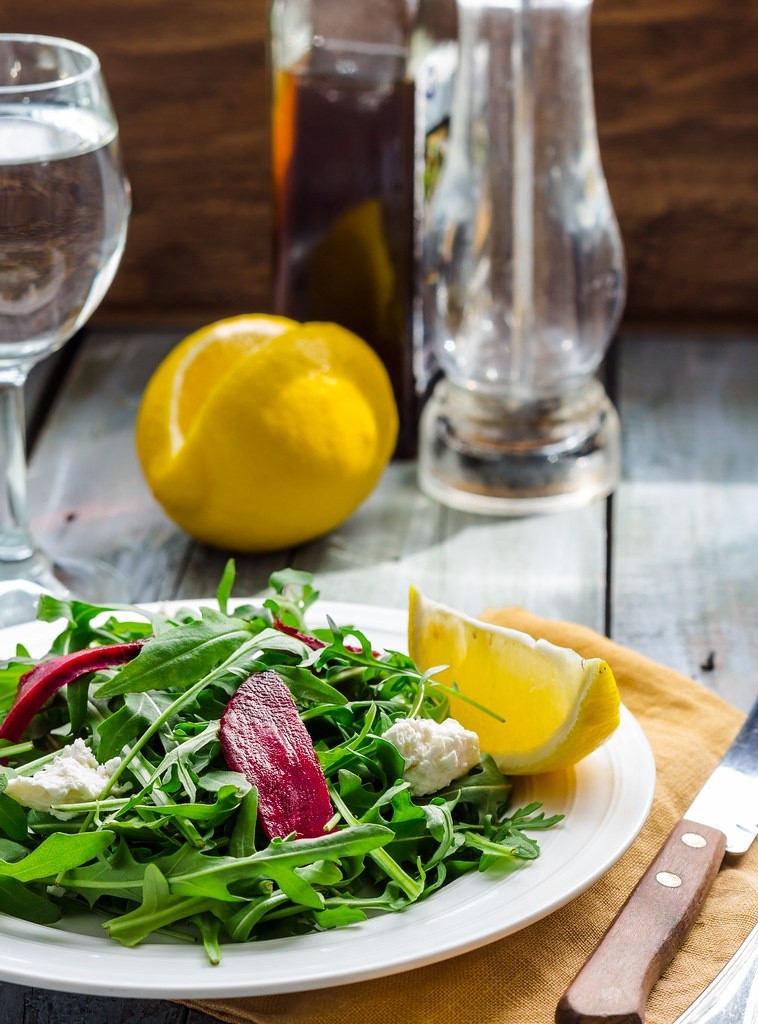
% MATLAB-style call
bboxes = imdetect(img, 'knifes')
[556,698,758,1024]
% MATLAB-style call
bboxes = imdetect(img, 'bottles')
[415,0,628,518]
[270,0,421,465]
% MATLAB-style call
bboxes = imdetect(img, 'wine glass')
[0,32,132,635]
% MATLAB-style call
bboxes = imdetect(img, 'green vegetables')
[0,558,565,964]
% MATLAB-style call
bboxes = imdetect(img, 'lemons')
[406,583,619,777]
[136,312,398,551]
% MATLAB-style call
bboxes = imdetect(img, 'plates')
[0,598,656,1000]
